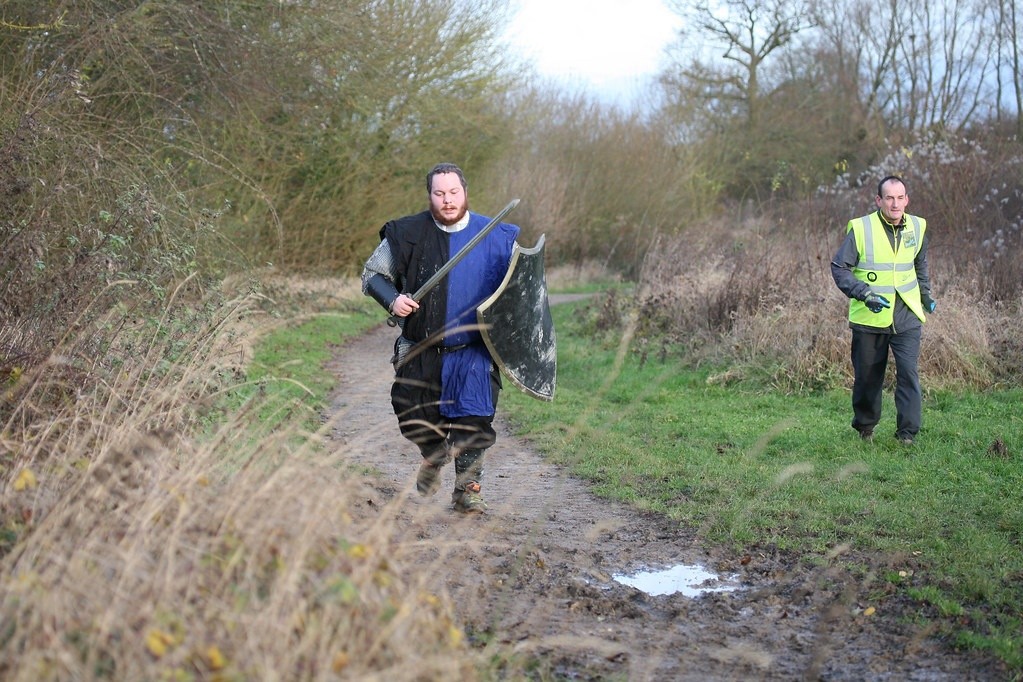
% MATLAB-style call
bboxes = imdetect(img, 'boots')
[451,447,486,514]
[416,439,451,496]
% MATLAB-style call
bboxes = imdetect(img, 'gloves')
[921,294,935,314]
[864,292,890,313]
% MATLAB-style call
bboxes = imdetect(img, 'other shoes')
[859,430,873,443]
[894,433,911,447]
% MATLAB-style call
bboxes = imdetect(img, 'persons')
[361,163,520,512]
[831,176,937,445]
